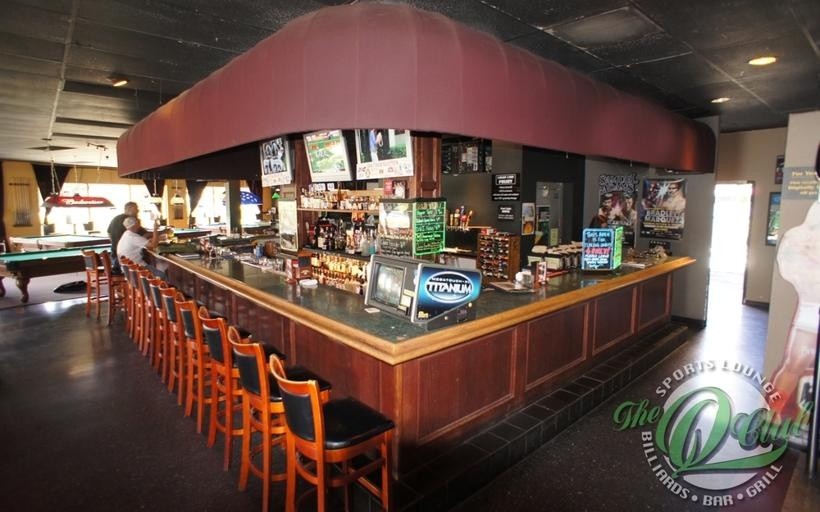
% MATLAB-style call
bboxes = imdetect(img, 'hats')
[123,216,137,228]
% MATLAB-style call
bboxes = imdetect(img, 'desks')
[0,234,112,302]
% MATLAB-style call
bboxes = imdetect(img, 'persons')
[597,191,616,225]
[614,191,636,226]
[588,214,608,228]
[641,181,686,212]
[369,128,382,162]
[116,216,169,284]
[106,201,172,275]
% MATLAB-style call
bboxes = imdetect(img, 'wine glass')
[205,243,214,260]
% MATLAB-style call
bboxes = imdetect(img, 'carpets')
[0,268,110,311]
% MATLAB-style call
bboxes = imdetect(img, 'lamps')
[39,142,116,209]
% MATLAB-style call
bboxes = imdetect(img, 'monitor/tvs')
[354,128,414,180]
[304,130,352,183]
[260,136,292,186]
[367,258,484,323]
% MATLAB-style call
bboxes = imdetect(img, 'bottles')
[300,182,382,296]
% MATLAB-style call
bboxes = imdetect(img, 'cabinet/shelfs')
[476,233,521,286]
[297,188,382,294]
